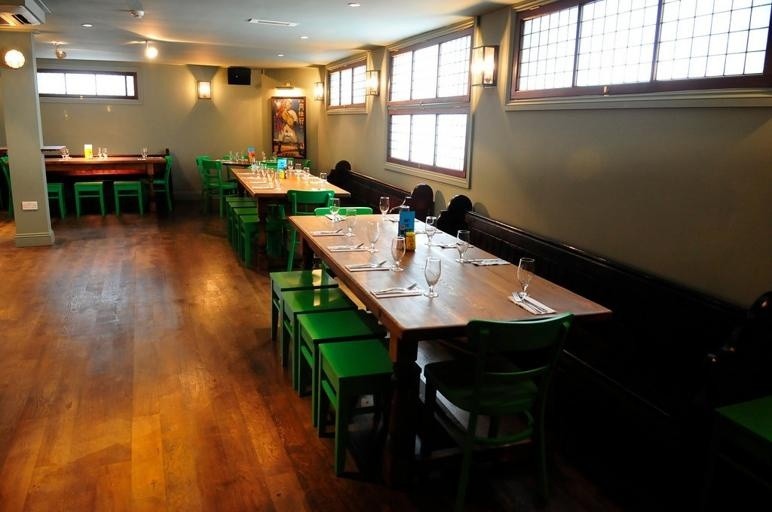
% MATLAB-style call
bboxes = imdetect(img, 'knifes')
[517,291,546,312]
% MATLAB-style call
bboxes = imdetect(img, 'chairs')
[153,156,173,213]
[0,154,15,221]
[418,308,574,510]
[196,156,237,219]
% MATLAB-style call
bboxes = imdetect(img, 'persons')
[278,98,299,145]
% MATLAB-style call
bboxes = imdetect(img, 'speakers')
[228,66,251,86]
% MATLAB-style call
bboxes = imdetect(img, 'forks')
[320,229,343,235]
[376,283,418,293]
[514,291,542,315]
[330,243,364,251]
[350,260,387,268]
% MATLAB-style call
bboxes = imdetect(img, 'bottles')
[279,169,285,179]
[405,232,415,251]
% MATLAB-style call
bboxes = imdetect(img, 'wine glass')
[391,236,405,271]
[287,161,328,190]
[379,196,389,224]
[345,208,357,237]
[425,216,438,249]
[60,147,72,160]
[327,198,340,224]
[516,258,534,299]
[97,146,108,159]
[247,159,282,191]
[424,258,442,299]
[366,219,380,252]
[225,150,276,164]
[456,230,471,263]
[138,144,148,159]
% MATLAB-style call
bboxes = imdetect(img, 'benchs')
[301,160,434,270]
[433,195,772,510]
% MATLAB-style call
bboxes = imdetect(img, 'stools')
[268,267,423,477]
[47,180,142,220]
